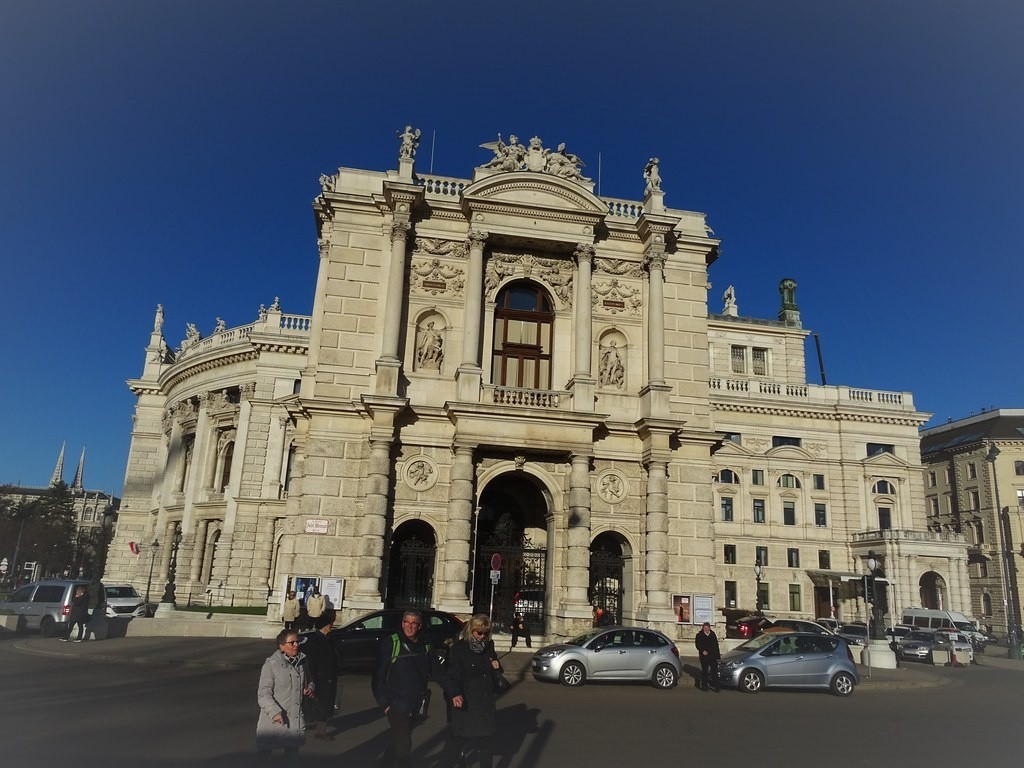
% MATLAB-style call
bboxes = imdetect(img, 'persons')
[284,591,300,630]
[511,613,531,648]
[695,622,721,690]
[725,285,736,306]
[481,133,591,183]
[602,341,622,384]
[307,587,326,630]
[10,574,24,590]
[418,321,441,367]
[60,586,89,642]
[303,614,338,742]
[255,630,316,767]
[186,297,281,344]
[644,157,662,194]
[434,614,504,768]
[155,304,163,332]
[397,126,418,158]
[592,606,613,642]
[373,609,434,768]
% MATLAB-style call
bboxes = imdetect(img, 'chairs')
[794,638,807,654]
[781,636,793,654]
[620,633,626,645]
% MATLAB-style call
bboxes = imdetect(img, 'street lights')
[158,524,182,610]
[145,539,160,602]
[753,558,764,620]
[868,549,887,640]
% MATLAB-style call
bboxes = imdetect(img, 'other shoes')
[58,637,67,641]
[73,638,80,642]
[709,684,719,691]
[700,683,706,689]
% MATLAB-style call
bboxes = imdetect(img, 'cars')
[294,606,468,676]
[978,629,999,646]
[708,615,868,697]
[531,625,683,689]
[884,623,975,663]
[87,583,147,620]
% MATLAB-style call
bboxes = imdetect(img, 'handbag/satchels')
[415,691,429,721]
[493,666,511,696]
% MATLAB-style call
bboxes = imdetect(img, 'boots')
[315,720,332,741]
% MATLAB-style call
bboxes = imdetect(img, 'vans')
[0,579,107,638]
[902,606,986,653]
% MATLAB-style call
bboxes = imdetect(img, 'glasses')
[476,631,489,636]
[283,640,297,646]
